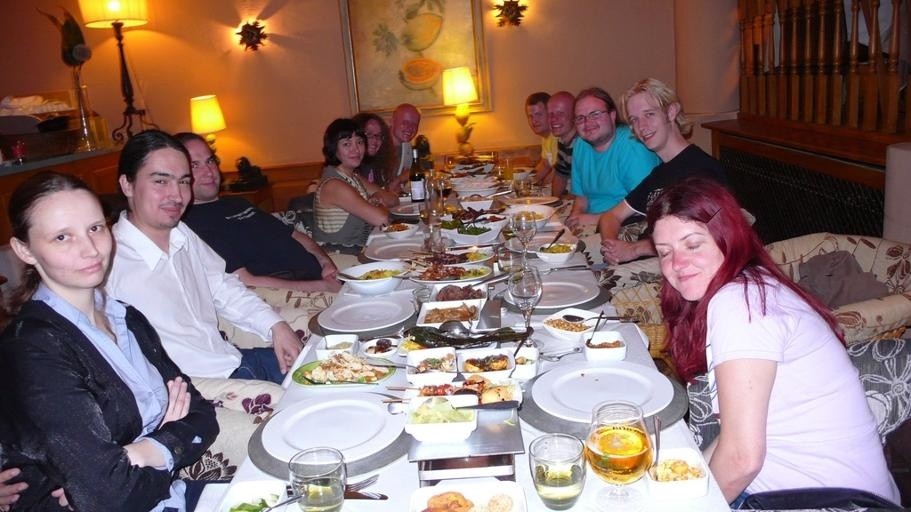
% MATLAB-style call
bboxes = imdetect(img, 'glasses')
[364,130,385,141]
[572,109,608,125]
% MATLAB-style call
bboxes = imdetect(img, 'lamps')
[76,0,159,144]
[237,22,267,53]
[189,94,228,183]
[439,66,478,155]
[493,0,530,29]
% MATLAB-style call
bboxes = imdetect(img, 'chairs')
[557,198,911,455]
[0,244,342,480]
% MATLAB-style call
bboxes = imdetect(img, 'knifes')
[288,485,388,502]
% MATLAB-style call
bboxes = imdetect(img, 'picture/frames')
[336,0,493,120]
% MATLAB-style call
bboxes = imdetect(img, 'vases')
[70,70,110,153]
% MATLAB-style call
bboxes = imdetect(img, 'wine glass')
[585,399,655,512]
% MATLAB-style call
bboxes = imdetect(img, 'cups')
[289,446,347,511]
[530,434,585,511]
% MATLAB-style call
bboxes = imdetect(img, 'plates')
[405,475,528,511]
[533,360,676,426]
[292,167,602,387]
[260,388,405,464]
[218,481,289,512]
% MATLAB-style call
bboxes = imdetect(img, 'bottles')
[409,145,426,202]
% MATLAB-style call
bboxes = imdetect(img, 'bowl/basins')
[404,307,630,444]
[646,445,712,503]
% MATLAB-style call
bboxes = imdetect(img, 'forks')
[269,474,380,511]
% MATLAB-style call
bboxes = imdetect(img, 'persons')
[548,90,579,201]
[101,130,307,387]
[1,442,70,511]
[648,176,902,509]
[593,77,733,264]
[172,133,345,292]
[351,113,416,192]
[1,169,230,512]
[526,93,557,184]
[312,118,402,247]
[563,88,663,225]
[386,104,423,182]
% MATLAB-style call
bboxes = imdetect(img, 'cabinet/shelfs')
[219,182,276,215]
